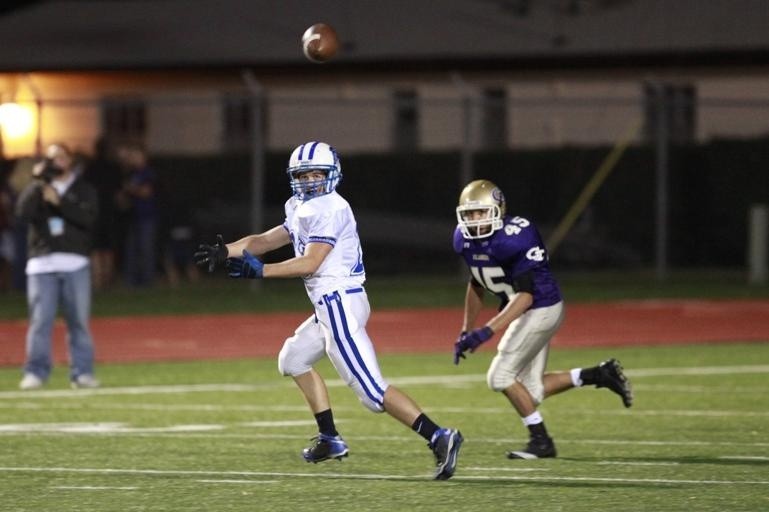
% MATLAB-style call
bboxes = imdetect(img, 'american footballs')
[302,22,340,65]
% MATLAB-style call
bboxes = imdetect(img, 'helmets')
[286,142,341,201]
[456,180,506,240]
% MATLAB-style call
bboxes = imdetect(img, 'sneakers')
[506,437,556,460]
[597,359,632,408]
[429,428,464,480]
[303,432,349,462]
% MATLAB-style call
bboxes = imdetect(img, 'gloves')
[455,326,493,363]
[193,234,229,273]
[226,249,265,280]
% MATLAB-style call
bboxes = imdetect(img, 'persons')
[194,141,463,480]
[2,136,158,289]
[15,142,101,389]
[452,179,633,459]
